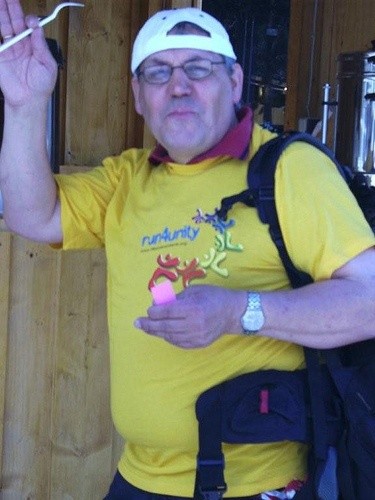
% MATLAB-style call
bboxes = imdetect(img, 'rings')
[3,33,16,38]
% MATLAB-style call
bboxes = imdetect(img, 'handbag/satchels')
[189,130,375,500]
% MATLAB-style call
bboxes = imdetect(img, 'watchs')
[240,291,266,336]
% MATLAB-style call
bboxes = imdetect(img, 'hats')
[131,7,237,74]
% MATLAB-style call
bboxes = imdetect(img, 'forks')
[0,2,86,57]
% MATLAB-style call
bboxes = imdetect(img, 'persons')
[0,0,375,500]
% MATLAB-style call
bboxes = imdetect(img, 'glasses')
[132,56,228,84]
